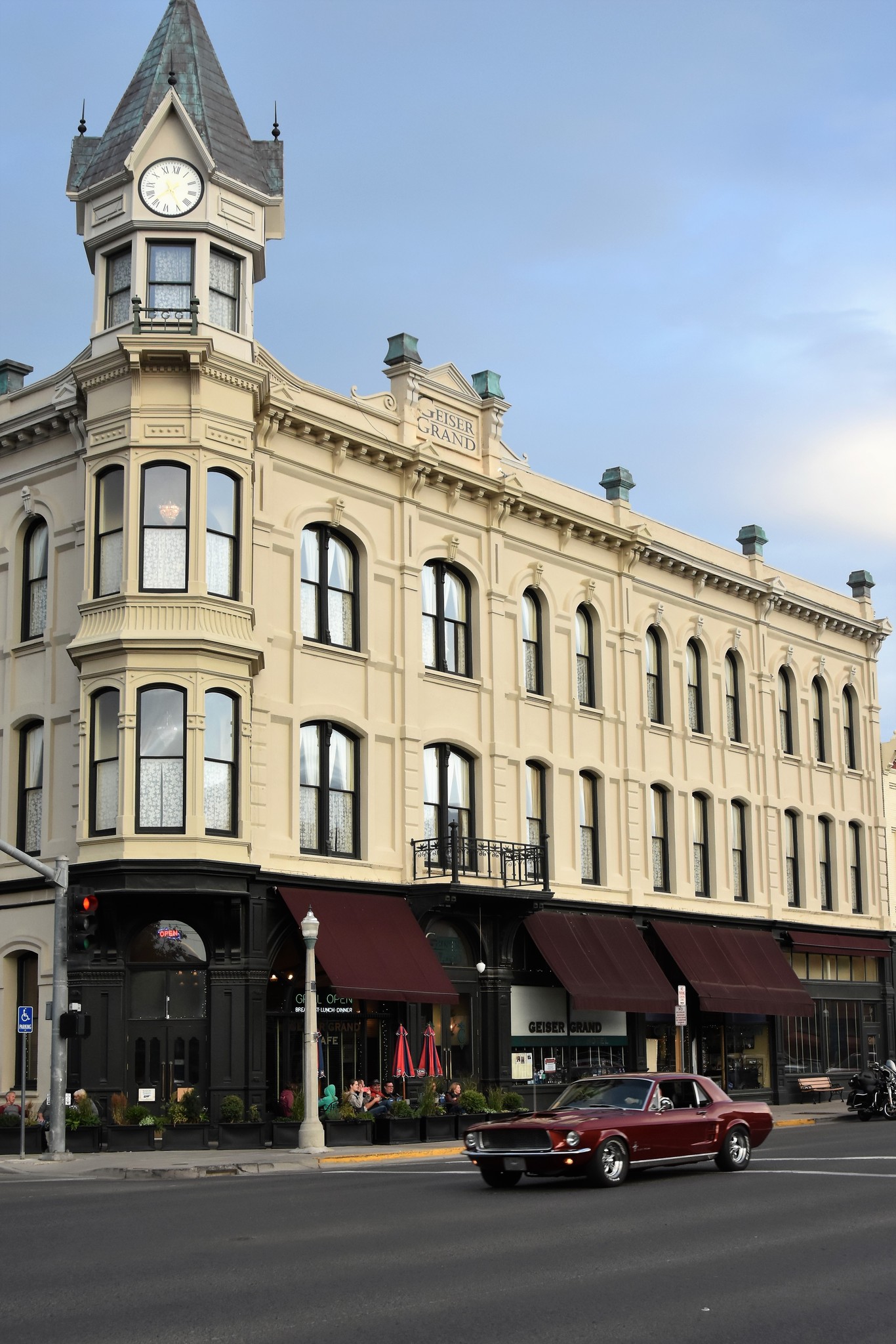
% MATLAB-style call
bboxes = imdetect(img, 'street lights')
[291,903,334,1154]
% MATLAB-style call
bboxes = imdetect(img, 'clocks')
[138,157,205,218]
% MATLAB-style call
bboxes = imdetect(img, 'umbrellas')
[391,1023,415,1101]
[417,1022,443,1083]
[315,1028,325,1096]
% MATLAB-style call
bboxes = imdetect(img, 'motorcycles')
[847,1059,896,1121]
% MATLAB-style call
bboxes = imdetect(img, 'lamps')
[497,467,507,478]
[158,499,180,525]
[476,907,486,973]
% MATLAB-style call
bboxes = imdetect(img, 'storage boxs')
[848,1077,857,1088]
[846,1090,865,1105]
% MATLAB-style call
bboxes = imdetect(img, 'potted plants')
[324,1085,375,1147]
[217,1095,267,1150]
[106,1091,166,1152]
[0,1100,44,1155]
[382,1075,534,1145]
[161,1084,210,1151]
[271,1085,305,1149]
[65,1092,102,1153]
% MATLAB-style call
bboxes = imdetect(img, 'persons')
[69,1089,99,1117]
[427,1081,447,1116]
[318,1084,338,1122]
[37,1087,69,1149]
[343,1077,401,1118]
[445,1082,467,1115]
[279,1082,299,1117]
[0,1091,30,1119]
[624,1085,673,1111]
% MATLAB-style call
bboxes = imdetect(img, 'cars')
[460,1071,773,1191]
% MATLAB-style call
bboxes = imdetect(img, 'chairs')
[323,1099,340,1115]
[93,1099,103,1147]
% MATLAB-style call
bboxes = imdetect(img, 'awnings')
[521,911,680,1015]
[273,886,461,1005]
[649,918,817,1019]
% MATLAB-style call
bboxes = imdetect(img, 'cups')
[389,1098,394,1102]
[441,1097,444,1103]
[397,1097,402,1101]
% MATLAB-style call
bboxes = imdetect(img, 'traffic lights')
[77,1015,91,1039]
[67,886,99,953]
[59,1013,85,1038]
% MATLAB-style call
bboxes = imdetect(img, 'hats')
[373,1080,380,1084]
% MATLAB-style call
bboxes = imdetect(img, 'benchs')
[797,1077,844,1104]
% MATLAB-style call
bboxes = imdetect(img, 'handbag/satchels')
[347,1094,365,1116]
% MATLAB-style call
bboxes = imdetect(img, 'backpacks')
[4,1105,19,1116]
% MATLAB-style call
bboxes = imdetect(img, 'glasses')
[386,1085,393,1087]
